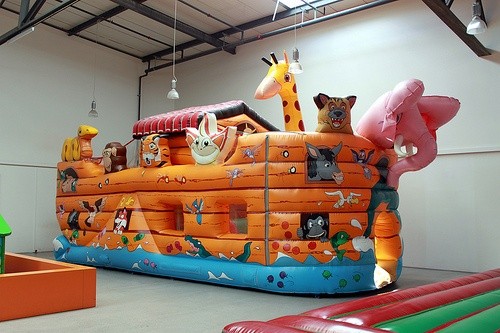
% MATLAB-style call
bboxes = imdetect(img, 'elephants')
[355,78,461,191]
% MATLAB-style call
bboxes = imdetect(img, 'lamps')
[89,20,98,116]
[466,0,487,35]
[6,27,34,45]
[290,7,302,74]
[167,0,179,99]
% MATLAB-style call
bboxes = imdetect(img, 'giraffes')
[254,49,305,132]
